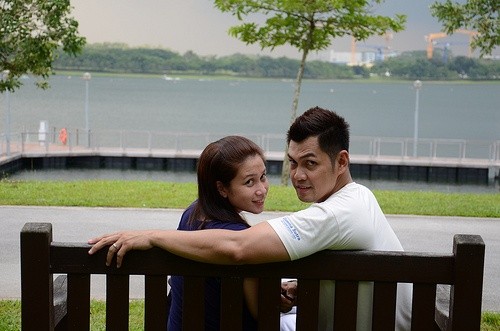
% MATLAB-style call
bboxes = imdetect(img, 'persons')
[88,105,413,331]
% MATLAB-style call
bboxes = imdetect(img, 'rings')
[113,244,119,250]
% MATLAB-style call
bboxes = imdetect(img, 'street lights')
[83,72,91,148]
[413,80,421,158]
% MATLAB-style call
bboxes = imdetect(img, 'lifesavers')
[59,129,67,144]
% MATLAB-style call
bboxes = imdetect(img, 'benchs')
[19,221,486,331]
